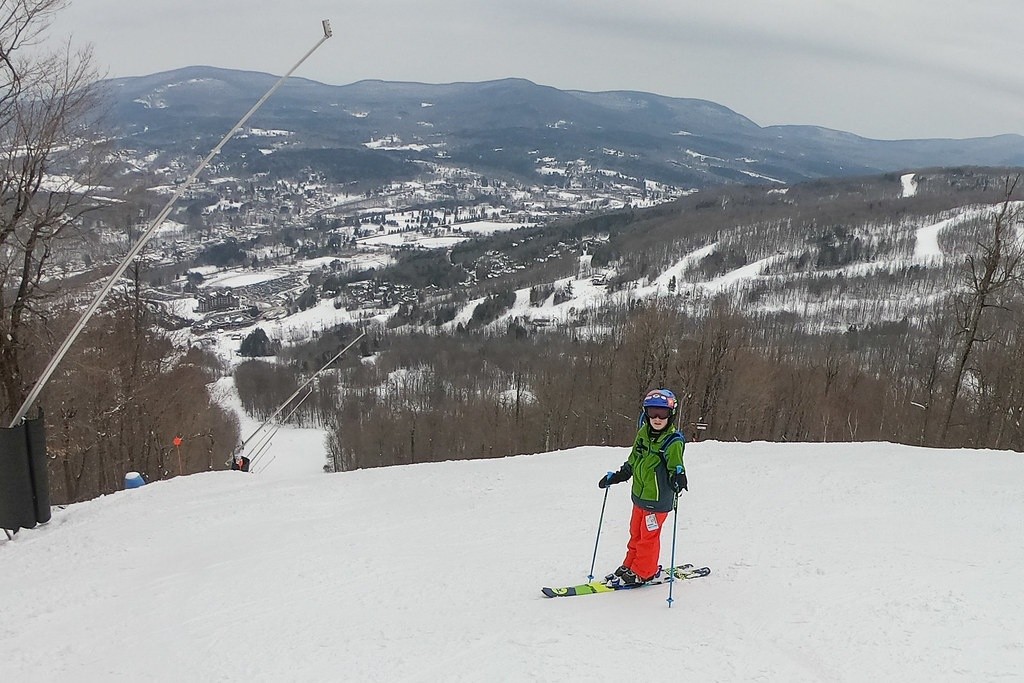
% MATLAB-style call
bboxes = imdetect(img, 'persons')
[599,389,688,588]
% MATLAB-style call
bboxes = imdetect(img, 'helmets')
[642,388,678,409]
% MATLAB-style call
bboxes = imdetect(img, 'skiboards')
[540,563,711,598]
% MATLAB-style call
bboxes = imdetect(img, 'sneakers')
[618,570,654,585]
[605,566,630,582]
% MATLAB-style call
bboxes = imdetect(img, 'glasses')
[645,408,669,419]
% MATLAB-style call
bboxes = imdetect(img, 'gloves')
[671,473,689,493]
[599,473,619,488]
